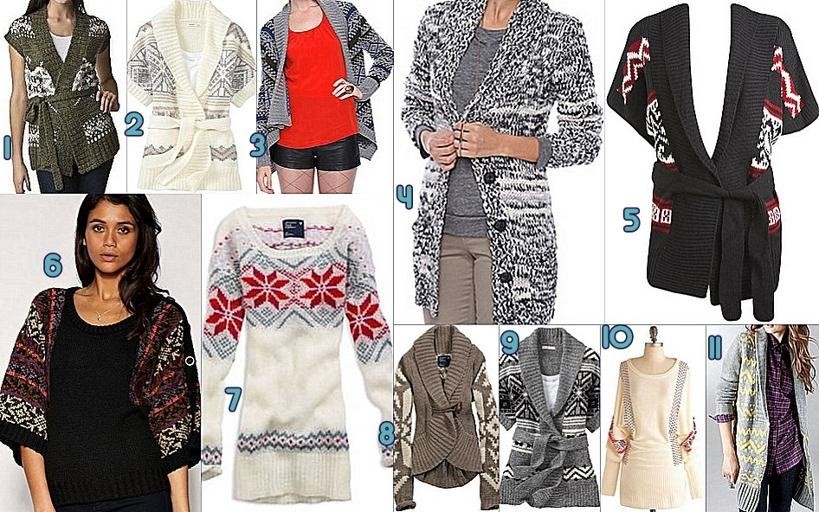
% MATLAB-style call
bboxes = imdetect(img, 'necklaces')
[84,285,123,321]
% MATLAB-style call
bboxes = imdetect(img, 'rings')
[345,84,354,96]
[458,122,464,159]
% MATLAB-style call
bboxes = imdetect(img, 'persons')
[707,326,818,512]
[602,325,705,511]
[398,0,605,324]
[3,0,122,196]
[256,0,392,192]
[0,197,200,511]
